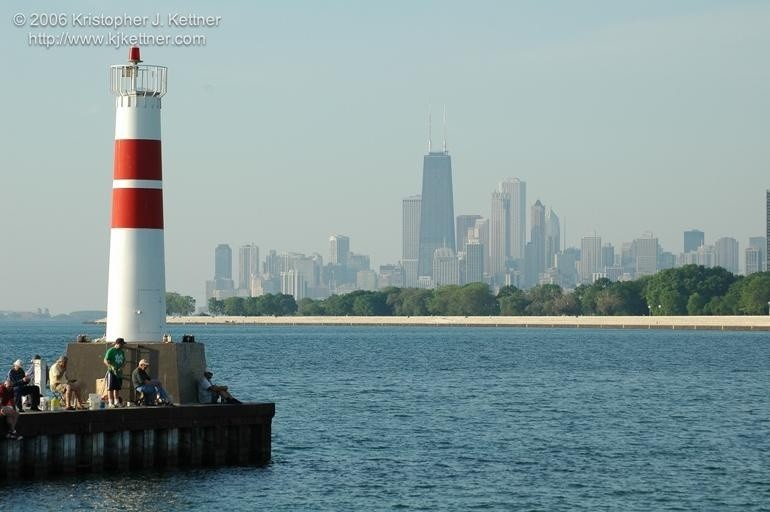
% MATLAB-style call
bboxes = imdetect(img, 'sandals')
[7,432,23,440]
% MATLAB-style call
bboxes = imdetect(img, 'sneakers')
[110,402,125,408]
[30,406,42,411]
[226,397,241,404]
[18,408,24,412]
[66,405,85,410]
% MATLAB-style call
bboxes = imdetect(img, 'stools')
[52,391,67,407]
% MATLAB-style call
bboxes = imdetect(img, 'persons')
[46,355,88,411]
[197,369,242,404]
[8,359,42,413]
[103,337,127,408]
[24,354,51,405]
[0,380,24,440]
[132,358,174,406]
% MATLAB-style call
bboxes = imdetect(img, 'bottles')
[100,397,105,408]
[163,333,172,344]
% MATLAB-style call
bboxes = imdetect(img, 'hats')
[204,367,214,374]
[139,359,149,366]
[116,338,126,344]
[14,360,23,367]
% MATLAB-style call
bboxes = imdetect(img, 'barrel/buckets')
[39,396,61,411]
[88,394,105,409]
